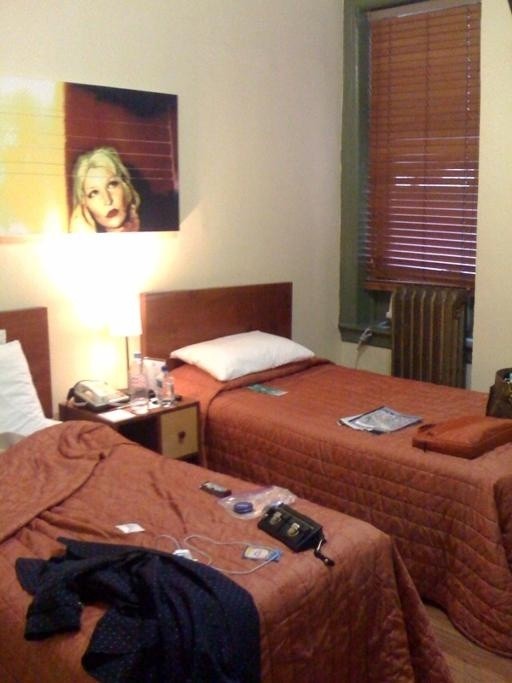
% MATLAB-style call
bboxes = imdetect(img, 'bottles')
[156,365,176,408]
[131,352,150,415]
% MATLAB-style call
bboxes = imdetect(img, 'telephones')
[71,379,130,411]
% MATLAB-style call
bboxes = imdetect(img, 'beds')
[0,304,460,683]
[133,280,512,663]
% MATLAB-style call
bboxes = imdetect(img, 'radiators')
[384,283,467,390]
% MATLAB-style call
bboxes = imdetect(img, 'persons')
[67,146,179,232]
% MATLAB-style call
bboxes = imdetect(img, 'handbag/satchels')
[257,502,336,567]
[410,415,511,460]
[485,368,511,417]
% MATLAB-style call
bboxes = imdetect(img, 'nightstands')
[57,383,204,469]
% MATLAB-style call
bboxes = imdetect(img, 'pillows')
[0,337,66,441]
[169,328,319,384]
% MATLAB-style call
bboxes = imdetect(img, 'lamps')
[107,296,145,400]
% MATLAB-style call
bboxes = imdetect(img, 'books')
[336,404,424,434]
